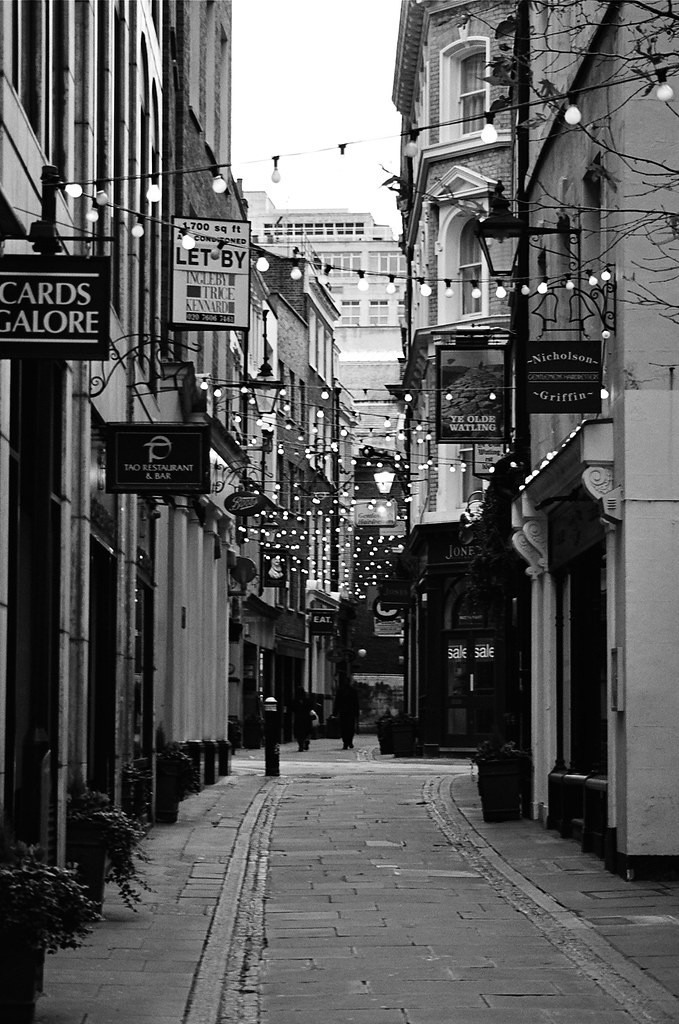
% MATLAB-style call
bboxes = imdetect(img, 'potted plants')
[469,738,531,824]
[0,840,103,1024]
[108,762,152,823]
[134,749,203,825]
[50,788,159,922]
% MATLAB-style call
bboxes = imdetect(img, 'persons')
[293,687,311,751]
[332,677,359,751]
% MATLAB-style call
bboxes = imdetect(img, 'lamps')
[212,358,286,414]
[472,178,581,277]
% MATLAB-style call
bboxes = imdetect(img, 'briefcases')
[326,714,343,739]
